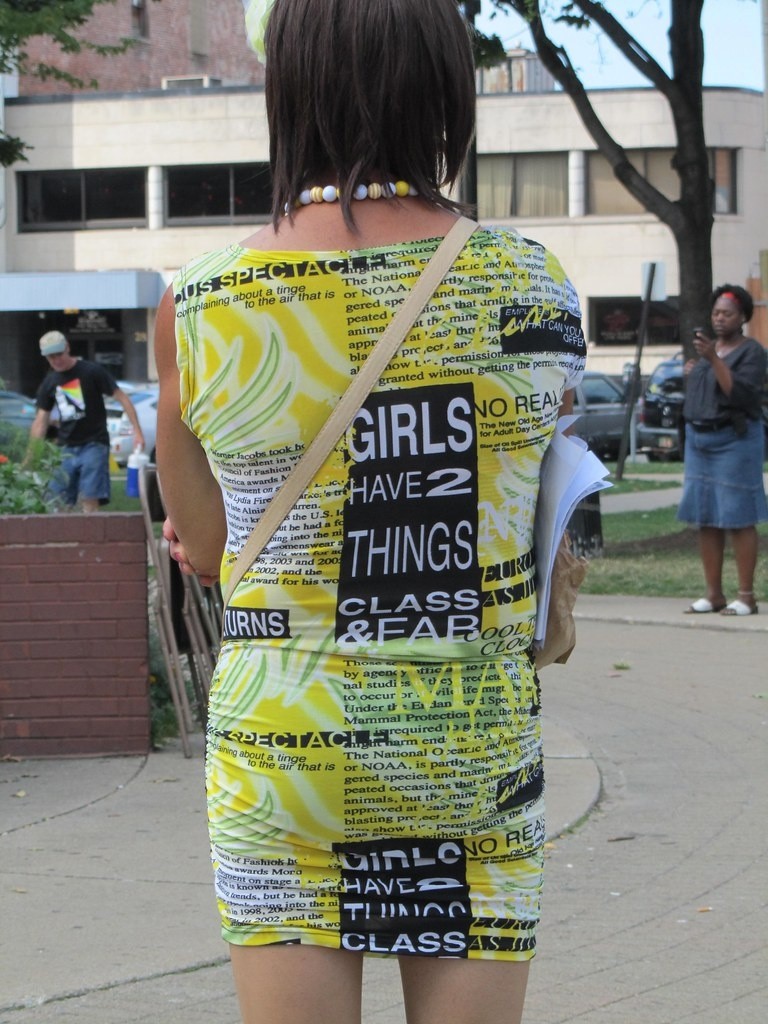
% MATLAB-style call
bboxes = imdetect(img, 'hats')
[39,331,69,356]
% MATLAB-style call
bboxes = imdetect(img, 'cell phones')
[694,327,703,340]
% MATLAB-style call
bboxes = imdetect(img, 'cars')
[0,389,37,432]
[571,370,627,446]
[102,379,161,467]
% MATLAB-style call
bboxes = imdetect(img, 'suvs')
[634,350,686,465]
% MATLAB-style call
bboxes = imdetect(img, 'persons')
[677,284,768,615]
[155,1,590,1024]
[16,331,145,512]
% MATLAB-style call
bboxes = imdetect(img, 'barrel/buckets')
[126,448,150,498]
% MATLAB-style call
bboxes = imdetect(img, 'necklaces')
[283,182,417,211]
[716,351,721,357]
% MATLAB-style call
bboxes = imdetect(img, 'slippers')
[720,600,759,615]
[683,594,727,615]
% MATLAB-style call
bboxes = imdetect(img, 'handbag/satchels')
[533,528,590,672]
